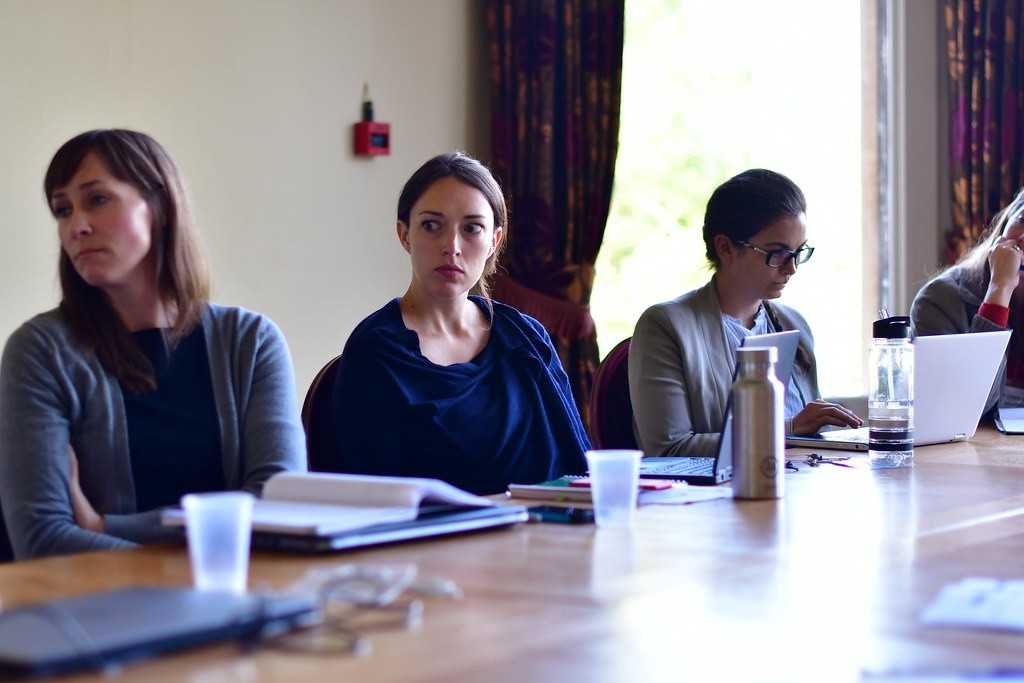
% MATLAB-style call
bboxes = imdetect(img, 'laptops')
[783,332,1011,453]
[585,330,800,485]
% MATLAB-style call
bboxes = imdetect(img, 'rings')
[1013,243,1021,252]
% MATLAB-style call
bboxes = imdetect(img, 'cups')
[585,451,641,525]
[182,494,253,593]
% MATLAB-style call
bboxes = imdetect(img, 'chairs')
[301,355,340,472]
[590,337,643,451]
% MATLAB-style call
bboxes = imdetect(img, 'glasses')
[729,235,815,268]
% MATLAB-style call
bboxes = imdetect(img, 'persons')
[626,166,864,457]
[0,126,308,562]
[909,187,1024,430]
[303,143,595,495]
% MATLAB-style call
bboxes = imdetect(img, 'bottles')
[867,316,915,467]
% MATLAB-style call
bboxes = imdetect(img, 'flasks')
[733,346,785,500]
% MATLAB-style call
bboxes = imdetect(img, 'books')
[507,474,736,506]
[160,468,532,554]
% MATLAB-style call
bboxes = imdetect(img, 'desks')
[0,420,1023,683]
